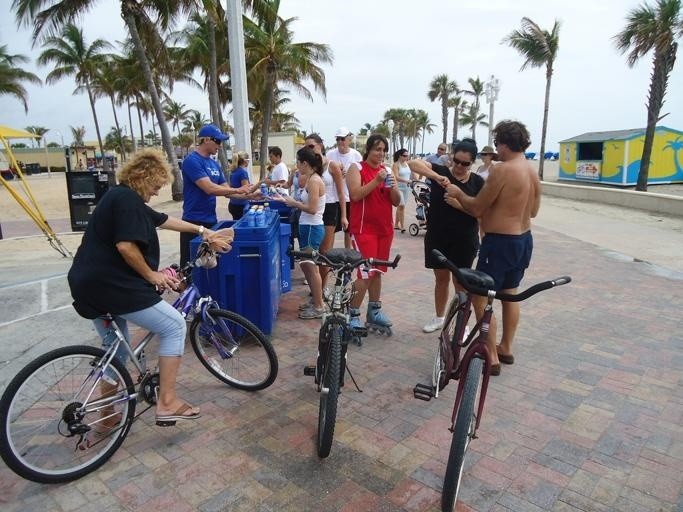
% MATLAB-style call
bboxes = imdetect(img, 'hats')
[335,127,350,138]
[478,145,498,160]
[197,122,229,141]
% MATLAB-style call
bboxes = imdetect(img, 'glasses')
[335,136,348,142]
[308,142,318,149]
[453,154,474,167]
[400,155,408,158]
[494,139,501,147]
[209,137,223,146]
[437,148,445,151]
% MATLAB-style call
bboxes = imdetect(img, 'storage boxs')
[187,192,296,350]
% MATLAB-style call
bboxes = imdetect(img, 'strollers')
[408,180,430,235]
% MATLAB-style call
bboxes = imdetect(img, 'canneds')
[385,174,392,187]
[262,182,267,197]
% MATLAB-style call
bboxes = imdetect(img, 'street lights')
[483,74,501,147]
[366,130,371,139]
[386,118,395,164]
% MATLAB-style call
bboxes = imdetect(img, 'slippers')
[481,363,500,377]
[154,403,203,422]
[497,354,514,366]
[98,411,124,437]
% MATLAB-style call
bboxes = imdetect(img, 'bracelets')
[198,225,205,235]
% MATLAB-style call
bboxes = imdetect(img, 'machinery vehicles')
[0,161,25,179]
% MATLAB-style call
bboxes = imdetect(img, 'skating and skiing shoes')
[365,300,395,337]
[346,307,367,346]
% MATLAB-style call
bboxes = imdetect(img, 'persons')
[176,124,267,276]
[294,133,350,300]
[407,137,486,335]
[271,143,329,319]
[443,119,544,378]
[345,133,405,335]
[230,126,499,246]
[66,144,235,437]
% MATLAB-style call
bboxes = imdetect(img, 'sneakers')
[297,307,327,320]
[423,316,445,334]
[298,298,314,311]
[458,325,470,345]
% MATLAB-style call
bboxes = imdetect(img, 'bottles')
[247,203,270,226]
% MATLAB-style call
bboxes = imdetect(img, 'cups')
[382,171,392,188]
[260,184,268,197]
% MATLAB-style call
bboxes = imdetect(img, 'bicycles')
[285,244,400,457]
[0,239,278,483]
[412,248,571,511]
